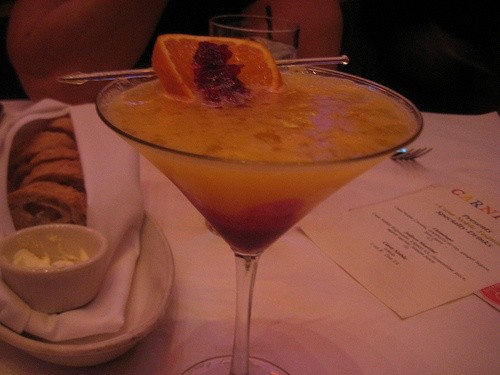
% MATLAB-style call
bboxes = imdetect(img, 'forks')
[392,147,431,160]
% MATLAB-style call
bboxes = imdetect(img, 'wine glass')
[95,66,425,374]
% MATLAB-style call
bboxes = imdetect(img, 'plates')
[0,101,176,366]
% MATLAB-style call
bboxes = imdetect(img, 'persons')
[0,0,343,106]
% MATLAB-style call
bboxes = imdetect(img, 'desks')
[0,110,500,375]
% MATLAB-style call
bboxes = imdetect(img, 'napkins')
[0,98,146,343]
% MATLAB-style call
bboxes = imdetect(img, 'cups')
[0,222,107,315]
[209,14,300,66]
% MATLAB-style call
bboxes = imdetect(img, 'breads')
[6,115,88,233]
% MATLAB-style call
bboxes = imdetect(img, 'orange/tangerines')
[153,33,283,98]
